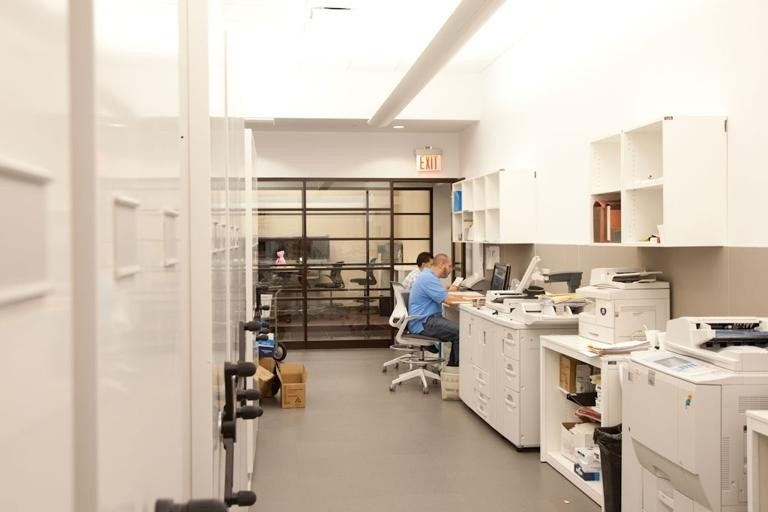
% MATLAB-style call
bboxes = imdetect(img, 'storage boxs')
[276,361,307,408]
[441,366,461,401]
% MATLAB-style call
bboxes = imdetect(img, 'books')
[593,199,621,243]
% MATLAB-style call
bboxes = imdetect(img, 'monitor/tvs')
[489,262,511,291]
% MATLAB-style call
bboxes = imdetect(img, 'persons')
[408,254,459,367]
[402,252,440,353]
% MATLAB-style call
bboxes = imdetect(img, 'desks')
[458,303,579,451]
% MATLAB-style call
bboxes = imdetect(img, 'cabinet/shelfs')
[588,115,728,247]
[452,167,536,244]
[539,332,653,511]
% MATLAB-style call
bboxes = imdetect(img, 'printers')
[576,266,672,345]
[621,313,768,512]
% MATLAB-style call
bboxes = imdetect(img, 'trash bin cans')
[592,422,623,512]
[439,364,460,401]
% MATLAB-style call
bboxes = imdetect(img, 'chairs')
[350,257,377,314]
[383,281,438,372]
[314,261,345,320]
[389,290,449,394]
